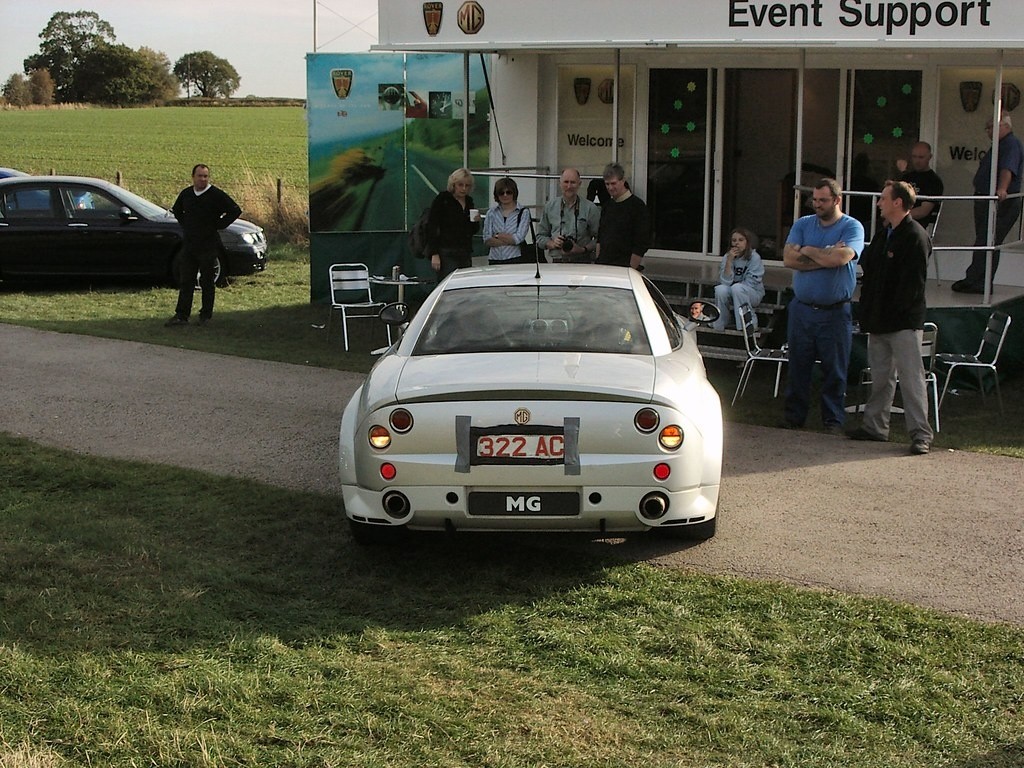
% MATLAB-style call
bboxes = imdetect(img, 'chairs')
[325,263,391,351]
[922,199,944,286]
[929,310,1011,417]
[896,322,941,433]
[730,302,790,408]
[777,172,815,256]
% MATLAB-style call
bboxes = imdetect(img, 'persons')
[951,110,1024,293]
[163,163,242,327]
[842,179,935,455]
[593,161,650,272]
[769,177,865,436]
[482,177,531,265]
[536,167,601,264]
[425,167,481,283]
[690,302,711,322]
[706,226,766,332]
[859,141,945,284]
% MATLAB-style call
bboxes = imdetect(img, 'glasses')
[498,191,513,196]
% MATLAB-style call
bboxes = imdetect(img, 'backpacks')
[409,192,446,259]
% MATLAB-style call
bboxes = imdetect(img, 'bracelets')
[798,245,804,252]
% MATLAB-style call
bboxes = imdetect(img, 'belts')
[800,297,852,310]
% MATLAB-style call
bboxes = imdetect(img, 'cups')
[730,245,740,259]
[469,209,479,222]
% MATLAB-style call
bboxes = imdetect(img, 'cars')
[0,175,267,290]
[0,167,96,210]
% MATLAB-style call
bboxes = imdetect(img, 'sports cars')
[337,262,723,539]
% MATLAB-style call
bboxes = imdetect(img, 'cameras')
[559,234,577,252]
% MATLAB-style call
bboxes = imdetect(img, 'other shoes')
[164,315,188,327]
[911,439,930,454]
[951,278,993,294]
[197,316,210,325]
[844,426,886,442]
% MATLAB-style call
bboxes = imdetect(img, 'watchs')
[494,232,499,239]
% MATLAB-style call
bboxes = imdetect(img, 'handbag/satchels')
[516,206,547,264]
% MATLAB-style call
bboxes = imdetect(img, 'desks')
[367,277,426,339]
[844,324,904,414]
